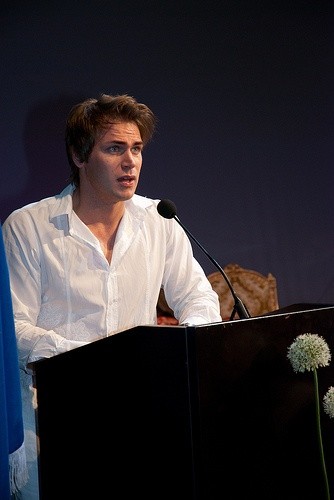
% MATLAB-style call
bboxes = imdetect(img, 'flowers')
[287,333,334,500]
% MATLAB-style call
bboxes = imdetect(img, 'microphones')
[158,199,251,320]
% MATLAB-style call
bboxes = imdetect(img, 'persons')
[2,92,222,500]
